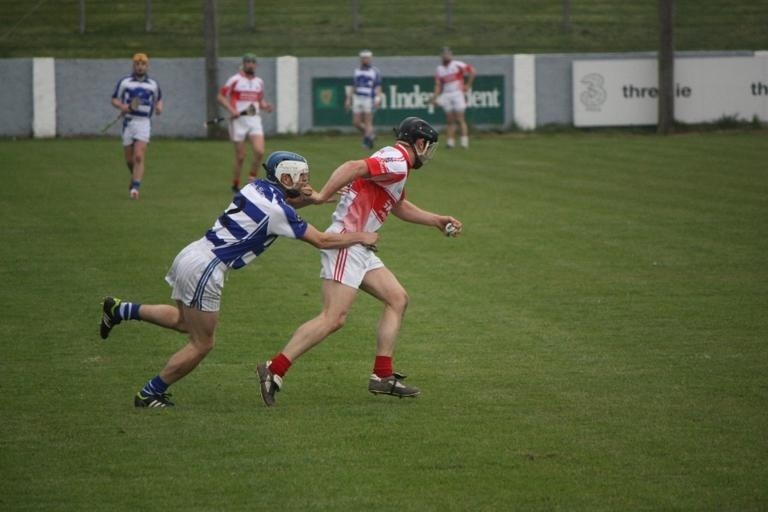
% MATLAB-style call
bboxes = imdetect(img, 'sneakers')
[233,185,240,192]
[135,391,174,408]
[369,372,421,396]
[130,187,140,199]
[257,360,284,407]
[101,295,122,339]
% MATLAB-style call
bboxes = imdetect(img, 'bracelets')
[375,96,381,101]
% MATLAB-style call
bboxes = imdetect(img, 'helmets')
[398,116,439,170]
[266,152,310,199]
[133,53,148,62]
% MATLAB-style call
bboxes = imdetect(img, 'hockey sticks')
[203,104,255,129]
[101,96,140,130]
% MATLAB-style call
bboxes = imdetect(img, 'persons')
[111,52,162,200]
[431,46,477,148]
[345,49,382,153]
[100,151,380,409]
[256,116,464,407]
[216,53,274,193]
